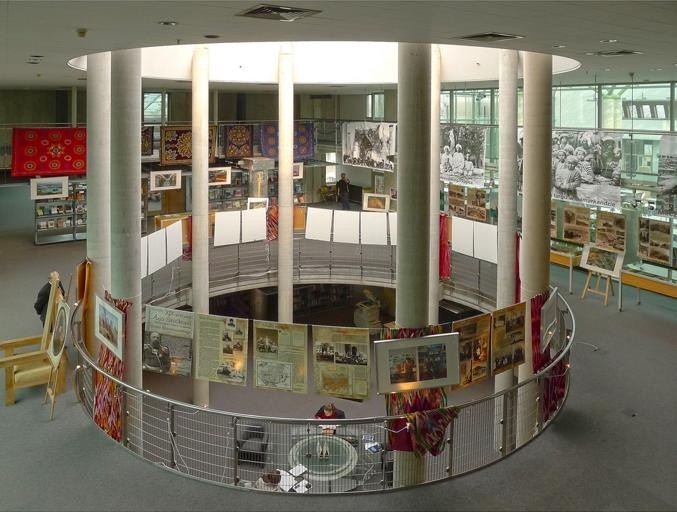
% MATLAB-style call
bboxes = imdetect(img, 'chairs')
[1,272,68,408]
[234,423,269,469]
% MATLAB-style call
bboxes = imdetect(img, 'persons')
[144,331,171,374]
[34,271,64,332]
[552,131,623,202]
[439,145,475,176]
[315,401,345,419]
[254,469,281,491]
[337,173,350,210]
[222,318,243,354]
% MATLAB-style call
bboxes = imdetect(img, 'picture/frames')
[538,287,559,355]
[149,169,181,192]
[247,197,269,211]
[45,296,70,371]
[579,243,624,277]
[140,124,253,164]
[362,188,397,213]
[292,162,303,179]
[374,332,459,395]
[30,176,68,200]
[90,293,126,364]
[208,166,231,185]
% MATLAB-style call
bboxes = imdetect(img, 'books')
[362,435,382,454]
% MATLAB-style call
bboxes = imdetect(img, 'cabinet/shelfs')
[31,178,87,246]
[446,185,673,269]
[196,169,306,206]
[252,284,353,320]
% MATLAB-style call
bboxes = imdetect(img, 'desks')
[286,434,358,495]
